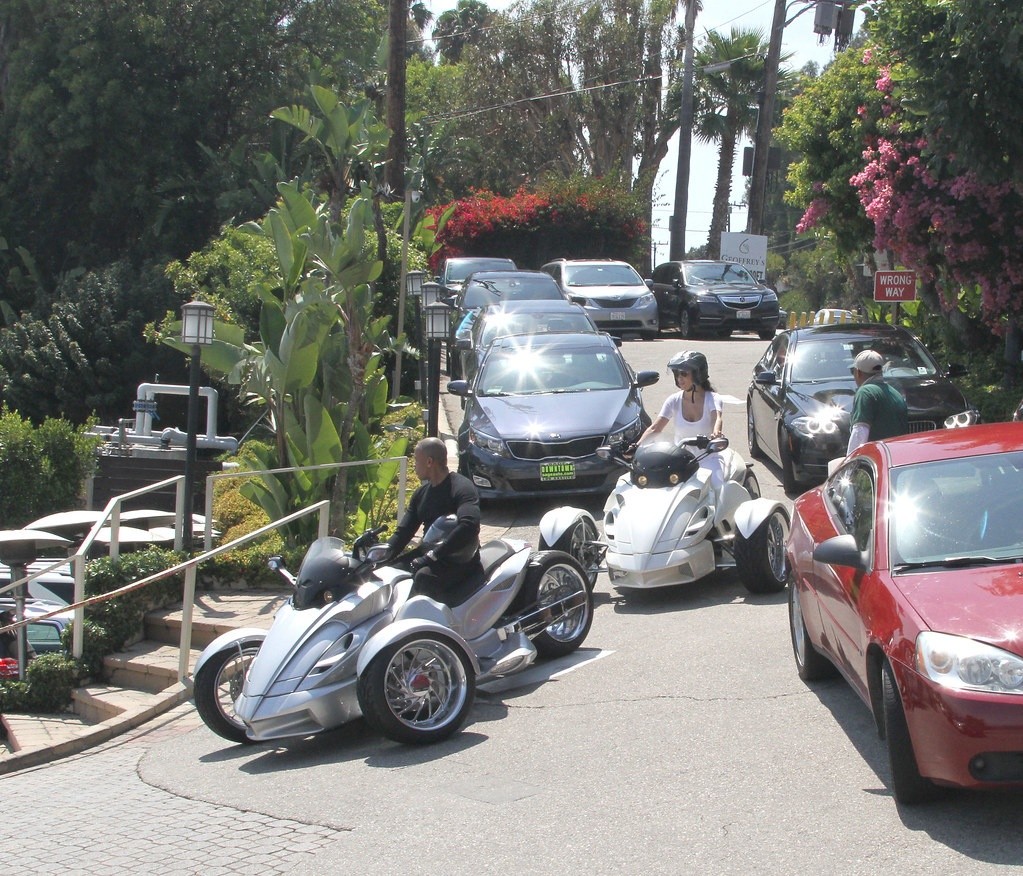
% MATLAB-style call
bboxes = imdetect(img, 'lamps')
[406,267,426,297]
[423,296,452,342]
[179,292,219,346]
[419,278,441,314]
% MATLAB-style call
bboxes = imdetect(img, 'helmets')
[419,515,481,574]
[667,351,708,386]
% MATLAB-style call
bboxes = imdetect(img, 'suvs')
[540,257,661,340]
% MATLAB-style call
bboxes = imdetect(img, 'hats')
[848,349,883,374]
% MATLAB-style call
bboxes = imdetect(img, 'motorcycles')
[594,434,793,607]
[191,517,602,750]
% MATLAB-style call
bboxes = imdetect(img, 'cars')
[453,298,623,404]
[456,272,564,322]
[0,557,78,696]
[640,259,786,340]
[442,330,663,505]
[782,419,1021,807]
[743,318,979,491]
[434,255,519,312]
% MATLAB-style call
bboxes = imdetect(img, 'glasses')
[850,368,854,374]
[671,368,689,377]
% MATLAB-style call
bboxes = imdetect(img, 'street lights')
[176,296,224,681]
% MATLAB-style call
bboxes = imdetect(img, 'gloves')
[409,554,434,574]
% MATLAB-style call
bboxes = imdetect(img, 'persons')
[848,349,909,456]
[385,437,480,611]
[624,351,725,512]
